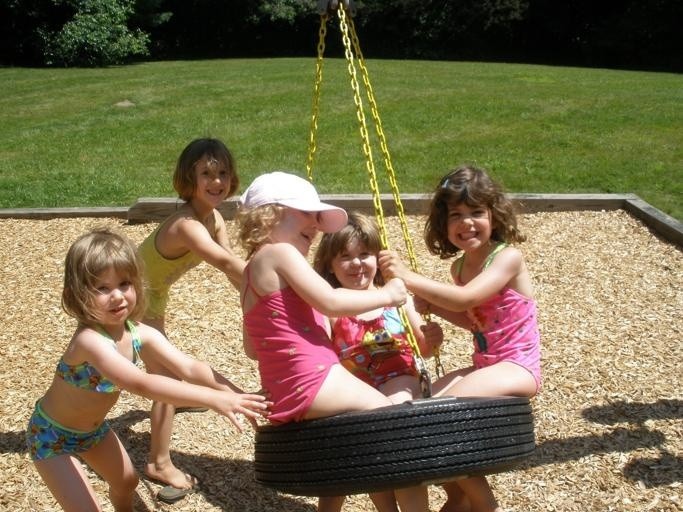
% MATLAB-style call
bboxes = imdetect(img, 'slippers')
[159,474,202,501]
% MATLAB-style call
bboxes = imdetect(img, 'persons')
[27,230,274,512]
[377,166,541,512]
[136,138,250,491]
[312,210,444,511]
[241,170,408,512]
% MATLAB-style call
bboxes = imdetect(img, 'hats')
[238,171,348,234]
